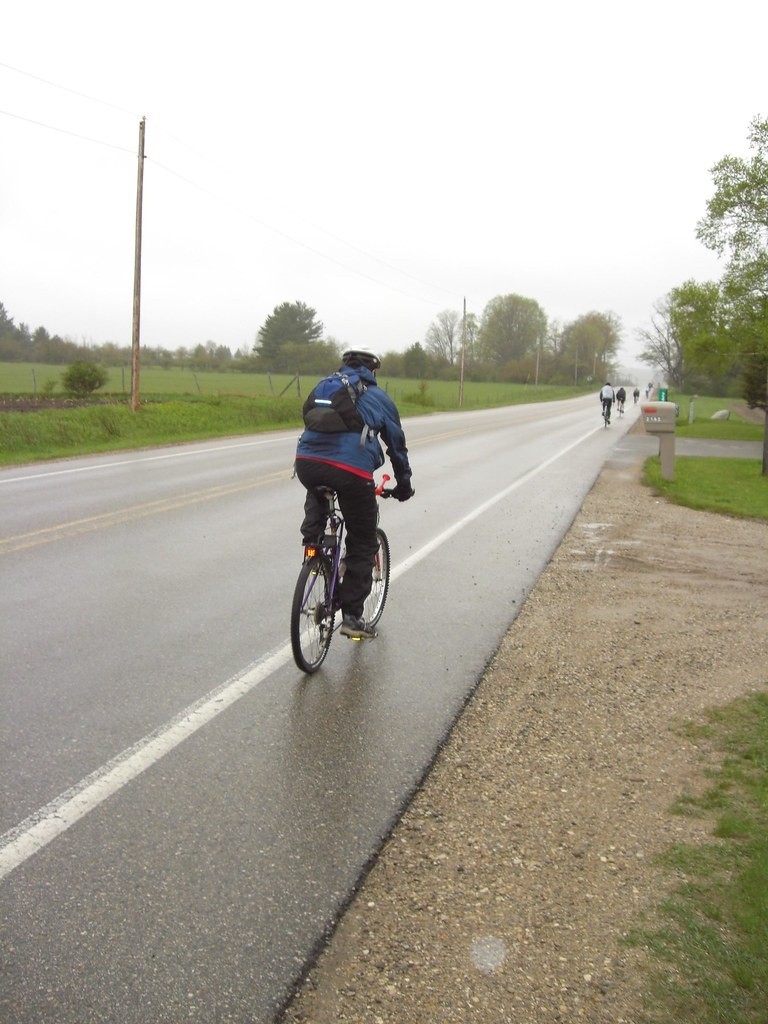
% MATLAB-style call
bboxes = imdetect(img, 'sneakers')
[340,615,378,639]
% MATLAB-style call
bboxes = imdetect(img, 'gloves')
[392,482,413,502]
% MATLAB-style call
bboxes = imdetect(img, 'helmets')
[342,347,382,368]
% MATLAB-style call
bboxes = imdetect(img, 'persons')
[296,346,413,637]
[633,382,653,400]
[600,383,615,424]
[616,387,626,414]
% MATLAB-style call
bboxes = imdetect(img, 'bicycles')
[290,474,413,673]
[617,396,625,416]
[601,397,613,428]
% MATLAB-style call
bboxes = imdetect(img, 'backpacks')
[302,369,376,434]
